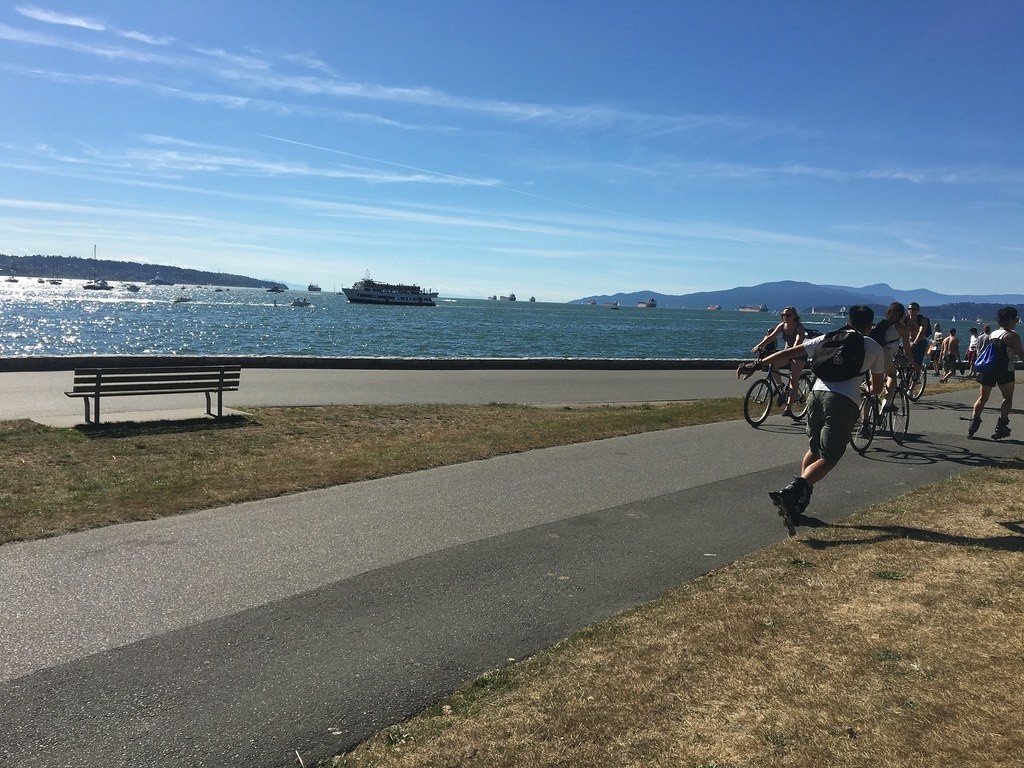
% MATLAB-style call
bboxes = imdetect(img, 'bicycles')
[884,352,927,401]
[742,349,812,426]
[849,364,926,453]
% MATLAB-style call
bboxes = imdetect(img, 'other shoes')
[782,409,792,416]
[938,379,944,384]
[932,371,940,377]
[884,404,899,412]
[878,414,883,426]
[776,389,787,407]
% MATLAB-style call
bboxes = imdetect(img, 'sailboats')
[82,244,111,290]
[5,252,19,283]
[37,258,63,285]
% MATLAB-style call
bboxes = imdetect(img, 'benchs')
[64,365,241,424]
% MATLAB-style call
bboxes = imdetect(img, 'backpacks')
[868,318,904,348]
[809,324,869,383]
[973,329,1015,373]
[917,314,932,337]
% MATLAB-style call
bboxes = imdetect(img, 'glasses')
[781,313,794,318]
[907,307,920,310]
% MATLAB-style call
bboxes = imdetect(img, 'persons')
[965,328,978,378]
[759,328,778,372]
[896,302,928,397]
[861,302,920,438]
[967,307,1024,439]
[929,324,943,377]
[735,305,885,536]
[751,306,808,416]
[938,328,960,383]
[976,325,990,360]
[929,324,943,376]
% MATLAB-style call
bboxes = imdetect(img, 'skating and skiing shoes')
[991,417,1011,439]
[768,476,809,537]
[791,482,815,527]
[967,417,983,440]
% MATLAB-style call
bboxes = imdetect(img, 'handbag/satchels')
[964,349,969,361]
[927,344,932,355]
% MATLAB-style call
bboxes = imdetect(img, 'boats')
[488,296,497,300]
[307,284,322,291]
[341,280,458,306]
[500,294,516,301]
[266,286,284,292]
[529,297,535,302]
[637,297,657,307]
[735,306,769,313]
[801,306,848,325]
[707,304,721,310]
[181,285,229,292]
[291,299,308,306]
[587,300,596,305]
[86,279,114,289]
